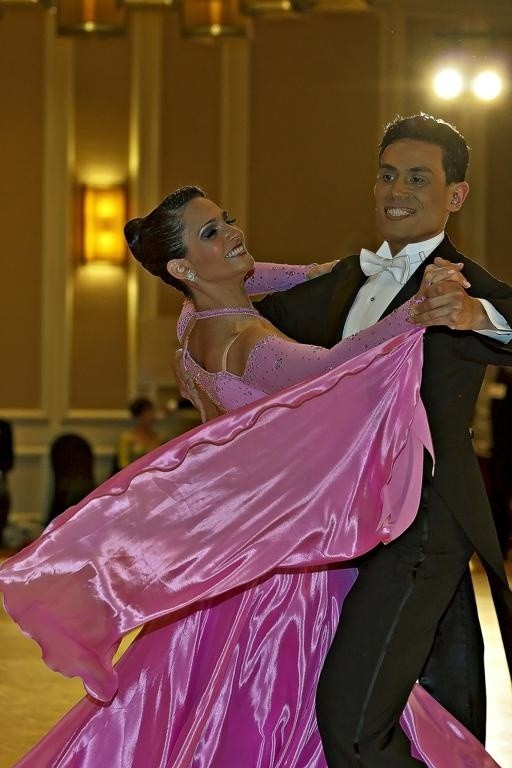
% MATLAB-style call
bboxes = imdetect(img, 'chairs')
[47,435,103,537]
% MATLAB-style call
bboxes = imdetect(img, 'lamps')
[78,180,132,272]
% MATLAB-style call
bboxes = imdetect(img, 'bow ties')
[359,248,410,284]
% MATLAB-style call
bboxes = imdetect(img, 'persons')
[0,419,18,549]
[162,396,204,448]
[169,104,509,768]
[115,398,172,471]
[0,179,509,768]
